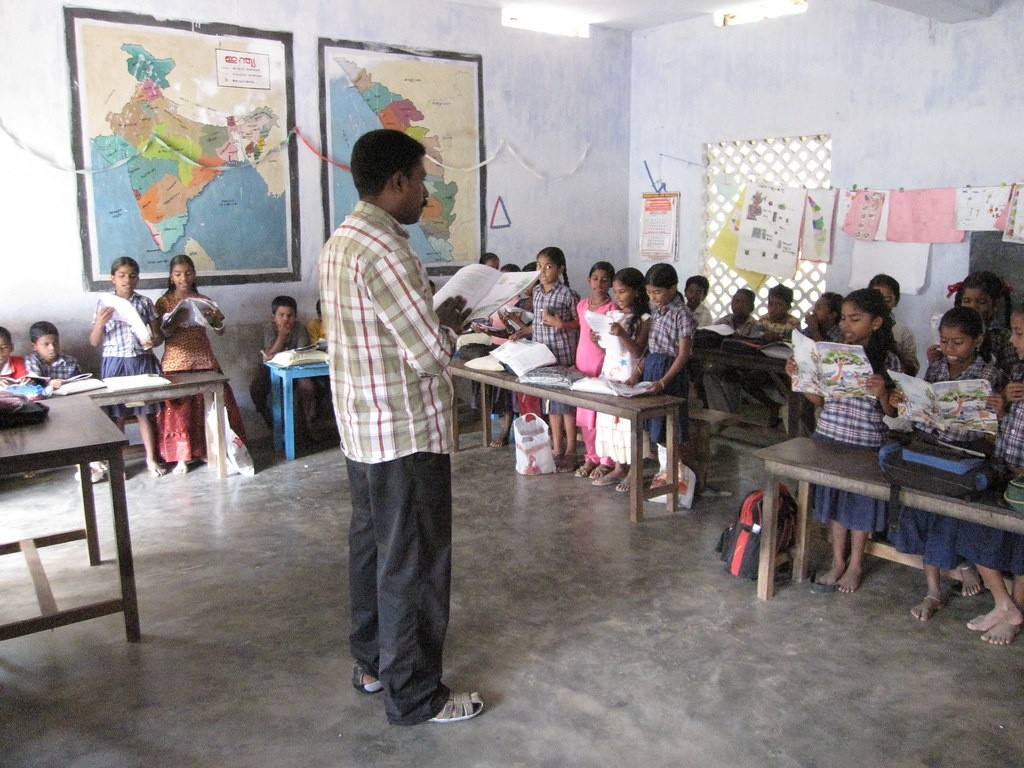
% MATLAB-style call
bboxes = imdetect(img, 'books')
[433,264,542,330]
[791,329,876,399]
[583,310,624,348]
[163,297,218,327]
[101,292,153,351]
[886,368,1000,435]
[489,338,557,377]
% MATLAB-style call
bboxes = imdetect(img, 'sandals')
[618,476,645,492]
[591,474,623,486]
[589,466,611,479]
[558,453,577,473]
[550,449,563,463]
[352,659,384,694]
[425,692,483,721]
[574,462,595,477]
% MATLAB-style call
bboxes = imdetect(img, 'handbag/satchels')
[0,389,49,426]
[513,411,556,475]
[269,349,330,367]
[205,401,254,477]
[647,442,696,509]
[5,382,47,401]
[1004,470,1024,514]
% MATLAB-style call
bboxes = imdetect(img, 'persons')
[249,296,328,443]
[479,247,695,493]
[89,254,251,477]
[0,326,36,478]
[685,272,919,433]
[24,321,109,471]
[318,129,484,725]
[892,270,1024,646]
[786,288,905,592]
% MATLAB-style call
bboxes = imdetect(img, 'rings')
[454,308,460,314]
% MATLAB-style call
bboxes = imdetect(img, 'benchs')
[682,405,734,494]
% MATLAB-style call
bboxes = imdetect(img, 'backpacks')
[716,484,797,580]
[877,426,1009,502]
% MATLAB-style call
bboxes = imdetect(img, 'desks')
[261,357,331,463]
[439,326,1023,601]
[49,367,231,480]
[0,396,141,645]
